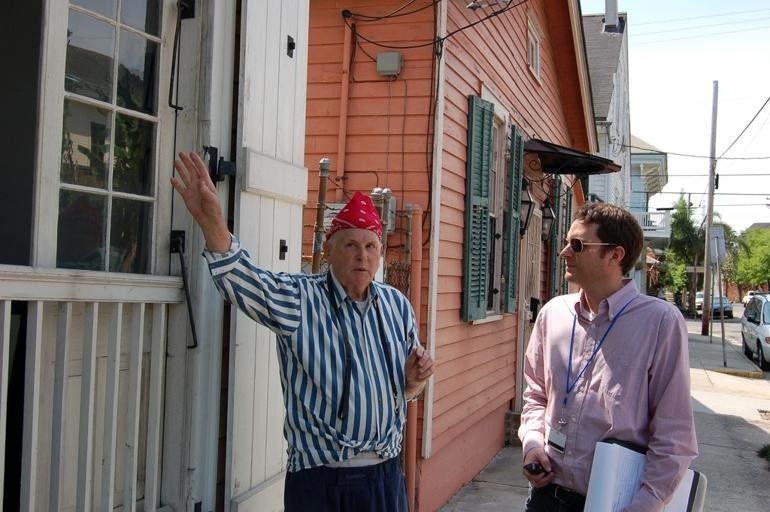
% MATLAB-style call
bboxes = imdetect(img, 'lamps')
[540,197,557,242]
[520,178,536,240]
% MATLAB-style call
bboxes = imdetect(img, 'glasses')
[563,238,612,253]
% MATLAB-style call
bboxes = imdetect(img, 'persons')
[516,203,698,512]
[169,150,435,512]
[673,288,682,308]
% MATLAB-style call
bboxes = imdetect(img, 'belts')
[554,485,582,505]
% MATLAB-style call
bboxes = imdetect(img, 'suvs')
[741,290,770,372]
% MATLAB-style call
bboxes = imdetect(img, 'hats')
[325,191,382,241]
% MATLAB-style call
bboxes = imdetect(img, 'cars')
[687,290,734,319]
